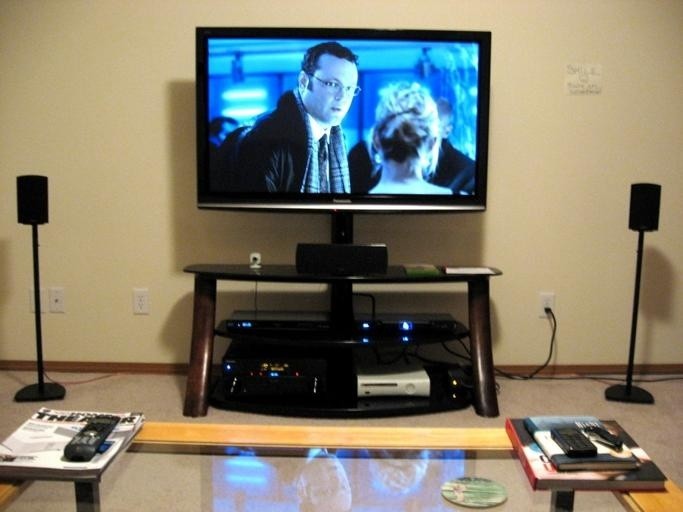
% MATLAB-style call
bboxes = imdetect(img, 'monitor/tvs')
[195,25,491,214]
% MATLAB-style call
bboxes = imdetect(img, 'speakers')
[294,242,389,277]
[629,183,661,231]
[17,177,48,224]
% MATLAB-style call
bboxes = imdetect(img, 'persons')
[207,38,475,195]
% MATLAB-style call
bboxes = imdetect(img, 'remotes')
[552,426,597,459]
[65,414,120,462]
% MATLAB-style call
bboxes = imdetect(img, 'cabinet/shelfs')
[182,261,502,419]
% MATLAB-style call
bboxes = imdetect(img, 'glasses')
[310,74,361,96]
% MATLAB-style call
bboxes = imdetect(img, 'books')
[0,403,146,479]
[504,412,669,492]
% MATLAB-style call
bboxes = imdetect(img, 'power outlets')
[131,288,150,315]
[536,292,556,319]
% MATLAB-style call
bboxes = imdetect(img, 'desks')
[0,421,682,512]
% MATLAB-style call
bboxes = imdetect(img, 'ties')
[319,135,328,193]
